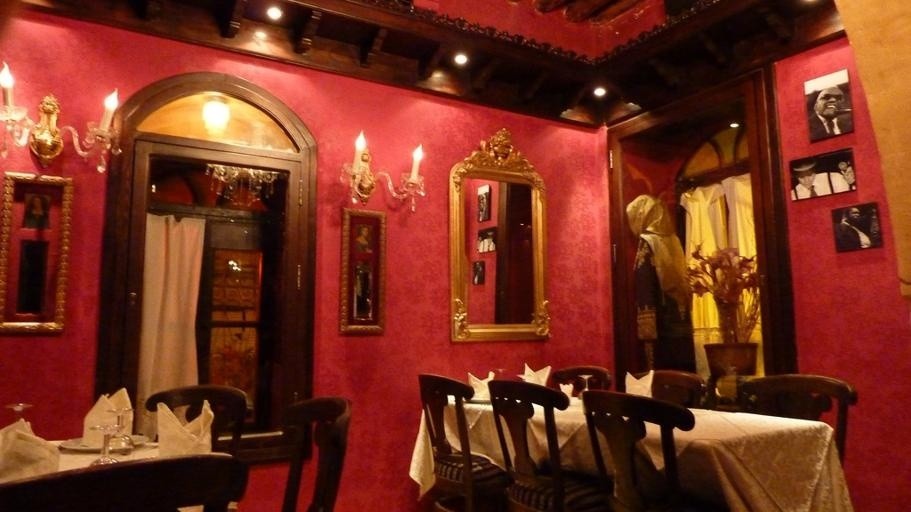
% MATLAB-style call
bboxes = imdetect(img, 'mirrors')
[450,128,555,342]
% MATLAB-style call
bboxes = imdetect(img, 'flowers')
[682,242,767,343]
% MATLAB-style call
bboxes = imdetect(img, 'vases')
[705,341,758,378]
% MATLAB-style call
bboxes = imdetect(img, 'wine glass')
[577,375,594,410]
[5,402,34,424]
[84,405,136,466]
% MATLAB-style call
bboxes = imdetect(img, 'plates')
[465,397,491,403]
[62,434,150,453]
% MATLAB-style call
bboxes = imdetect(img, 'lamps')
[339,128,427,212]
[0,61,123,173]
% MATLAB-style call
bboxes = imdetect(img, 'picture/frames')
[0,171,74,334]
[339,210,387,336]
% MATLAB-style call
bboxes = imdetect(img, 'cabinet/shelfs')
[147,202,268,439]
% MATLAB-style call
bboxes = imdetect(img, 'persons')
[478,234,483,250]
[479,228,496,252]
[18,192,50,312]
[352,222,377,323]
[807,84,854,143]
[472,261,486,284]
[789,158,855,200]
[837,206,878,251]
[477,193,490,221]
